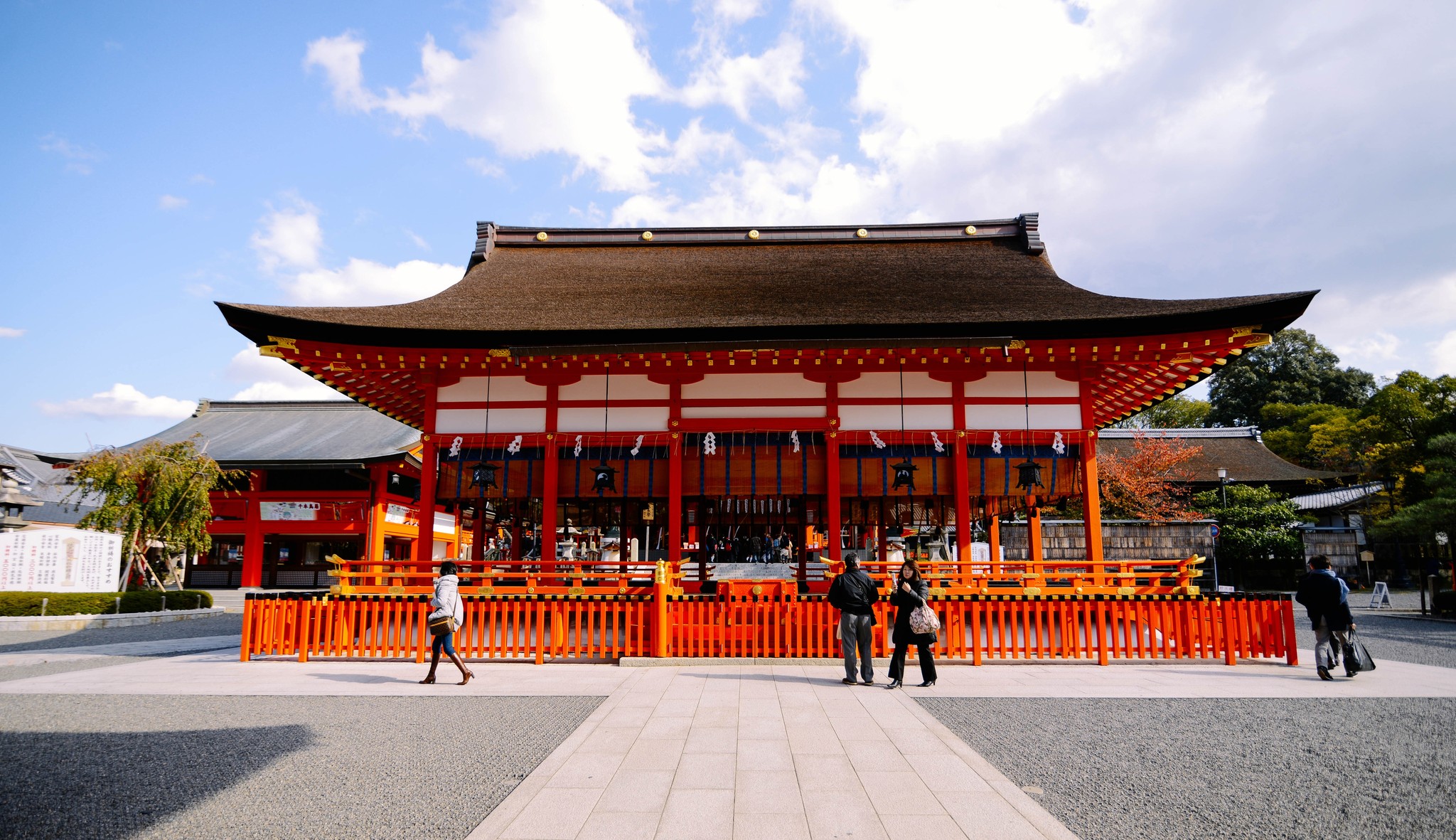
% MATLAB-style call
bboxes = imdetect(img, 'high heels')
[887,678,902,688]
[917,680,936,687]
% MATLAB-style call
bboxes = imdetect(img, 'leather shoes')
[1335,661,1340,666]
[1327,659,1336,669]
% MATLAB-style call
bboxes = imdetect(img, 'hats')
[1306,557,1311,564]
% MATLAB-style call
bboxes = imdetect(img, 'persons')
[706,526,798,566]
[494,528,541,559]
[886,560,938,689]
[1319,557,1350,668]
[1295,553,1355,680]
[417,561,476,686]
[134,551,187,590]
[829,552,881,684]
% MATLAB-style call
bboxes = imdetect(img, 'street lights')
[1214,467,1229,508]
[532,497,538,557]
[979,508,984,532]
[1378,473,1400,515]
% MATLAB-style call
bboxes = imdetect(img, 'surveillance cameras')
[1002,344,1009,357]
[514,354,519,367]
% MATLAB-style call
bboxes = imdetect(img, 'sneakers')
[1346,672,1359,678]
[864,680,874,686]
[842,678,858,685]
[1317,666,1334,680]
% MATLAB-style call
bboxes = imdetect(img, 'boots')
[419,653,440,684]
[450,652,475,685]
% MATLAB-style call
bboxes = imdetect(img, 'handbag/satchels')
[870,608,877,626]
[429,616,454,636]
[909,597,940,634]
[1343,640,1375,672]
